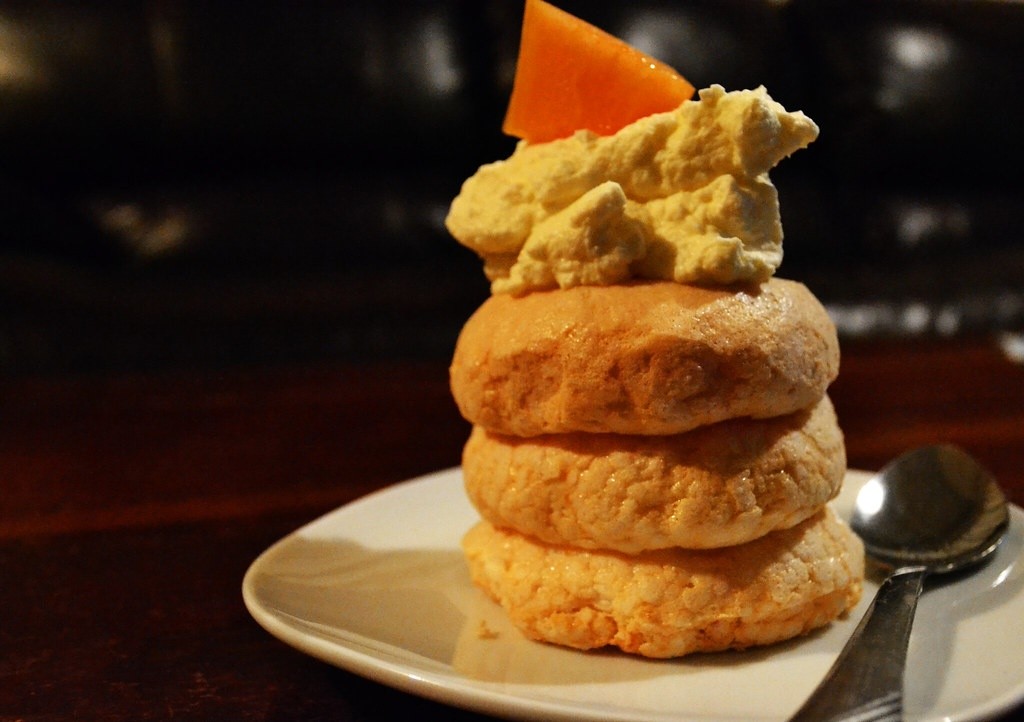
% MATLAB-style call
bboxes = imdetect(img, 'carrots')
[501,0,695,140]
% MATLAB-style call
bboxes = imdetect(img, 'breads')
[448,275,867,660]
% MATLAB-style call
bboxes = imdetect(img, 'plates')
[242,467,1024,721]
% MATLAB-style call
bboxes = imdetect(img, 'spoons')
[787,439,1010,722]
